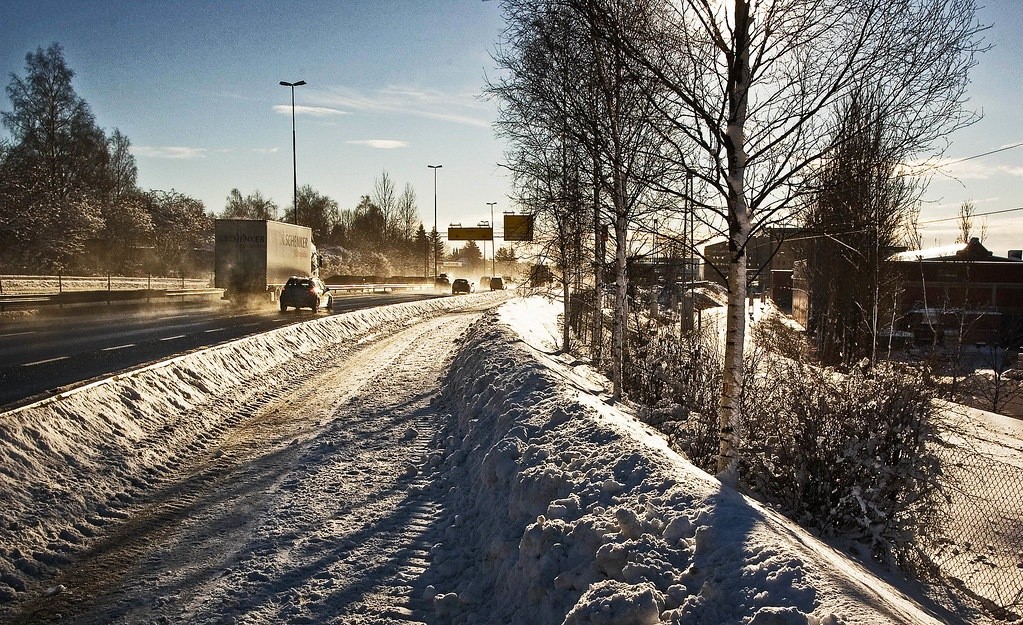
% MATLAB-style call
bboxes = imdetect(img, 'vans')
[503,276,516,285]
[481,277,493,288]
[490,277,508,290]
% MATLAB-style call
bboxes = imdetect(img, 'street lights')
[280,80,307,224]
[487,201,498,276]
[427,165,442,278]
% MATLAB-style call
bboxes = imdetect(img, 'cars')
[451,278,476,294]
[435,273,452,291]
[280,276,333,314]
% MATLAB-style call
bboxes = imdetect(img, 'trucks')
[530,266,554,287]
[213,218,324,307]
